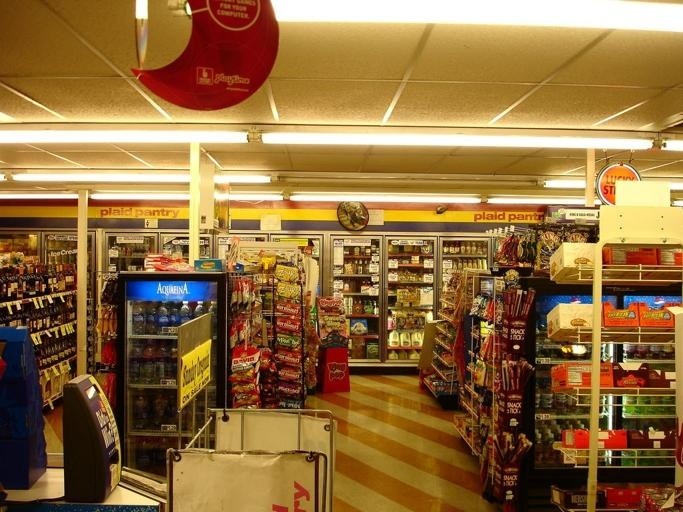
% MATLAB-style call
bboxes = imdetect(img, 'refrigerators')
[515,275,683,510]
[117,266,234,475]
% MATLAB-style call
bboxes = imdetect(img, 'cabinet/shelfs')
[0,227,683,512]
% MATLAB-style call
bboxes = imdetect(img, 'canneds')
[601,342,675,362]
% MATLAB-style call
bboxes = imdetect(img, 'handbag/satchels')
[318,331,349,393]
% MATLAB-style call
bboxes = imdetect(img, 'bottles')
[0,263,76,300]
[534,419,608,463]
[32,332,79,370]
[132,299,217,337]
[336,257,379,275]
[444,241,488,270]
[129,340,177,383]
[334,277,379,293]
[0,300,77,333]
[342,296,380,315]
[132,388,192,432]
[340,241,379,255]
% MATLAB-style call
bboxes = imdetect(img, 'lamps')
[0,0,683,206]
